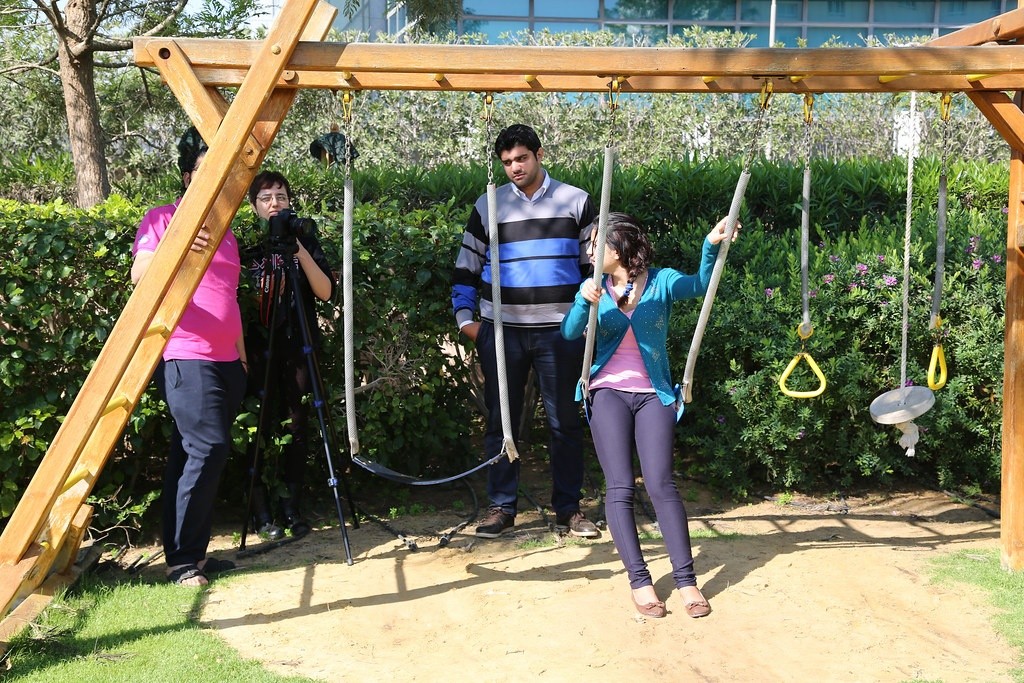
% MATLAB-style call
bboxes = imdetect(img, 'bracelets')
[241,361,246,365]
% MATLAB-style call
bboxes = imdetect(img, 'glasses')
[256,195,289,203]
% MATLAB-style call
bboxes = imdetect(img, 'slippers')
[166,563,209,588]
[200,557,235,574]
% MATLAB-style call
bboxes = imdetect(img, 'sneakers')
[555,509,597,536]
[476,507,514,538]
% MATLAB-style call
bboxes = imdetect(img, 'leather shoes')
[255,512,282,540]
[282,510,310,538]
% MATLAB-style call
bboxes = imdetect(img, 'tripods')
[240,239,358,565]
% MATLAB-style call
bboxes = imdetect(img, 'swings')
[342,83,519,489]
[580,75,774,431]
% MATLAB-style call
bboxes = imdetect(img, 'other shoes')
[631,589,665,618]
[680,585,710,617]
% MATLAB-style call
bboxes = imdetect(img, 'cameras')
[270,208,318,239]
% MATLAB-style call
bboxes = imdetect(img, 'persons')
[453,124,598,537]
[561,211,742,618]
[239,171,334,538]
[130,147,249,586]
[178,123,208,173]
